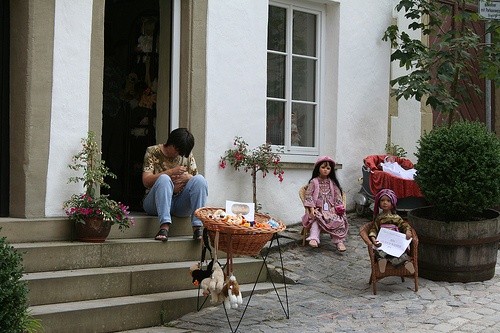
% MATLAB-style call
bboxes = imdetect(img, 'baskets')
[194,206,286,256]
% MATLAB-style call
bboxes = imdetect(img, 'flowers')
[61,130,135,233]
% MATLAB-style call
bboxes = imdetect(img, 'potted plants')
[407,120,500,283]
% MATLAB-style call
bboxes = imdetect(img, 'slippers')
[155,229,169,241]
[193,229,203,239]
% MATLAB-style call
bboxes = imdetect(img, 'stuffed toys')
[189,256,243,310]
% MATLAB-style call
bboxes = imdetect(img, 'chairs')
[360,221,419,295]
[299,185,347,247]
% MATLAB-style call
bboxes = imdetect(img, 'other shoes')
[405,262,415,274]
[308,240,318,247]
[336,242,346,251]
[378,259,387,273]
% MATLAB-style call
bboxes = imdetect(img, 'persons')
[383,156,397,165]
[301,156,348,253]
[369,189,415,275]
[143,128,208,242]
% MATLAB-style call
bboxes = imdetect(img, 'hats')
[378,189,396,204]
[316,156,335,167]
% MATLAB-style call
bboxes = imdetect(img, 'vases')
[74,214,111,243]
